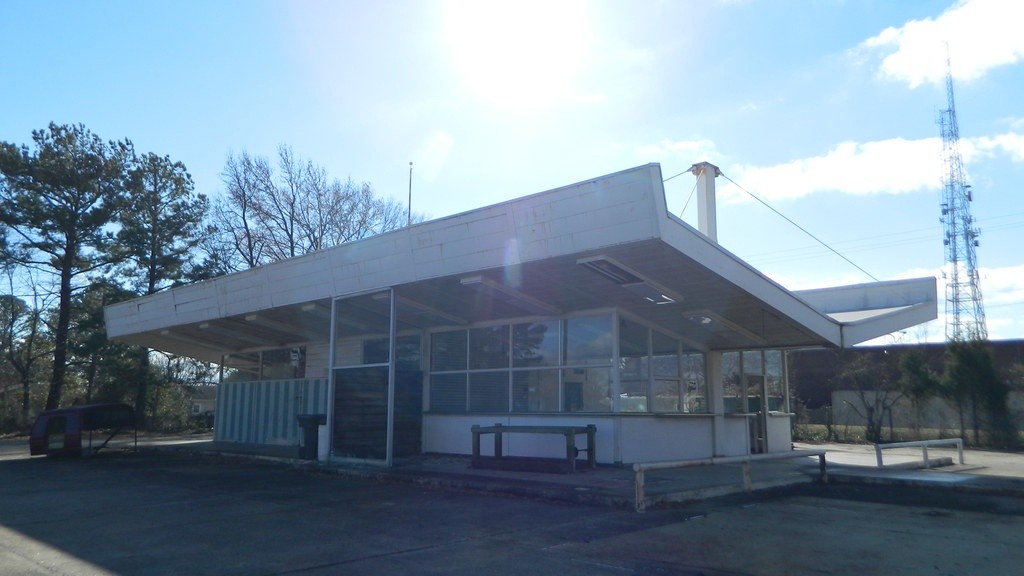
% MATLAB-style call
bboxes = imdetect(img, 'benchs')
[469,423,598,473]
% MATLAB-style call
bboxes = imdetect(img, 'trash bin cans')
[295,413,328,460]
